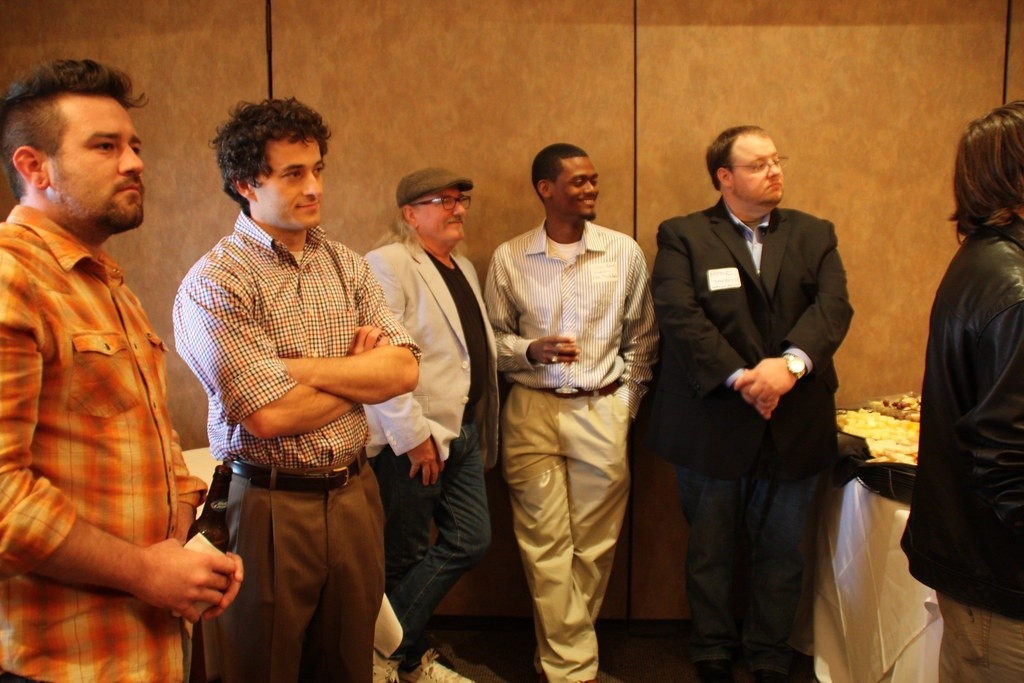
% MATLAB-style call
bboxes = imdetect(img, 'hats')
[396,168,474,207]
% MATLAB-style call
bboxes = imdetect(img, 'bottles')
[186,465,233,556]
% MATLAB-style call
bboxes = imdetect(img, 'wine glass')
[556,329,579,394]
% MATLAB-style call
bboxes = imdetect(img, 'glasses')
[409,195,470,210]
[727,157,789,171]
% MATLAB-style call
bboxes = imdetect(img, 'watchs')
[783,354,807,379]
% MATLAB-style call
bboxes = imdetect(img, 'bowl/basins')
[868,390,921,421]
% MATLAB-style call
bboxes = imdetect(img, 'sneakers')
[373,649,402,683]
[398,648,474,683]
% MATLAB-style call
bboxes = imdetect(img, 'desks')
[785,474,945,683]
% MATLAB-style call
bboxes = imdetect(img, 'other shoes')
[697,658,734,683]
[753,669,788,683]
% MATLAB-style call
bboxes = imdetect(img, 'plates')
[838,414,918,439]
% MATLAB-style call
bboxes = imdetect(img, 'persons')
[650,128,853,683]
[0,59,245,683]
[349,163,500,683]
[900,100,1024,683]
[172,100,423,683]
[479,144,660,683]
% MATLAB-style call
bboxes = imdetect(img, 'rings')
[551,357,559,364]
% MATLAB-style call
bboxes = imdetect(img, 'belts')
[541,379,620,398]
[231,449,366,490]
